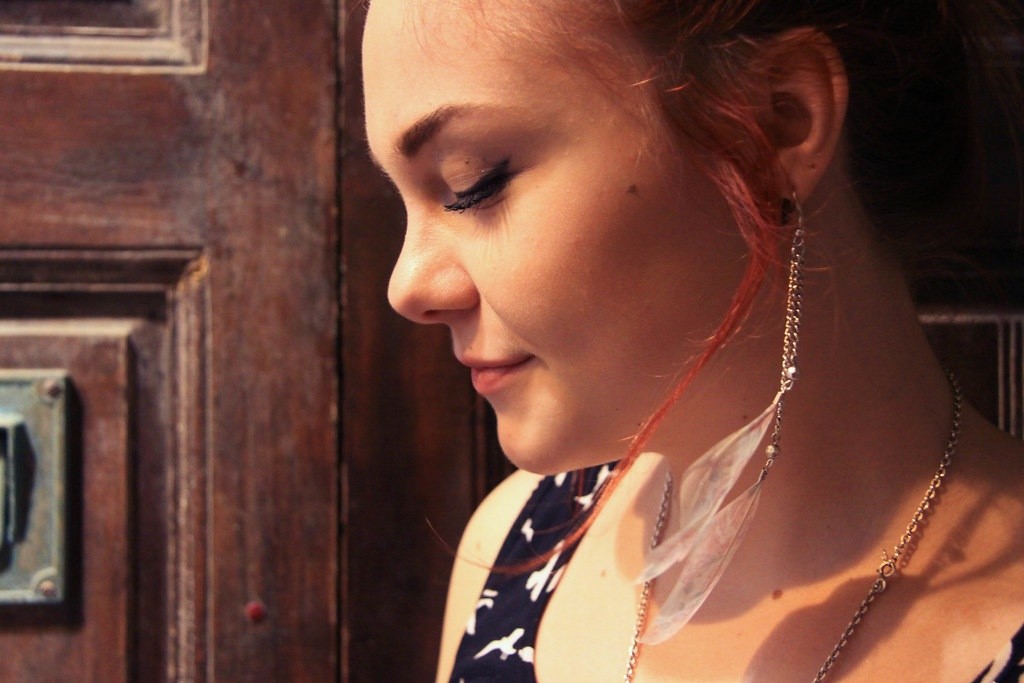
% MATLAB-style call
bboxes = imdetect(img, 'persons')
[360,0,1024,683]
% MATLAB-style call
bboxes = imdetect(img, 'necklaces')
[624,365,963,683]
